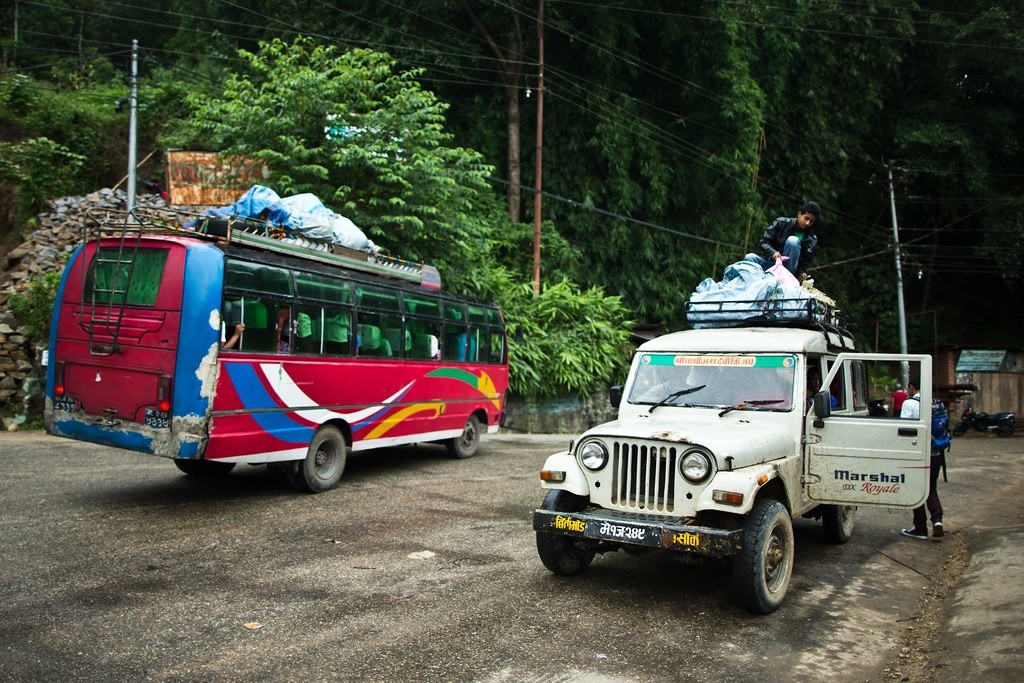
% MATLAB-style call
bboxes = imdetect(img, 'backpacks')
[909,396,952,452]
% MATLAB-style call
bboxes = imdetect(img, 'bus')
[43,206,512,495]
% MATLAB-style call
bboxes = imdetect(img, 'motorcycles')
[952,403,1016,438]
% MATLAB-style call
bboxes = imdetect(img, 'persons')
[744,201,821,285]
[892,384,908,418]
[900,379,944,539]
[221,321,245,350]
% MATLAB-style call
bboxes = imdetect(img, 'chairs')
[223,298,484,373]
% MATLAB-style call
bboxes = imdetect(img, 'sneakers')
[901,525,929,540]
[932,519,945,538]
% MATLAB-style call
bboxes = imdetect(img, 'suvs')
[532,298,933,615]
